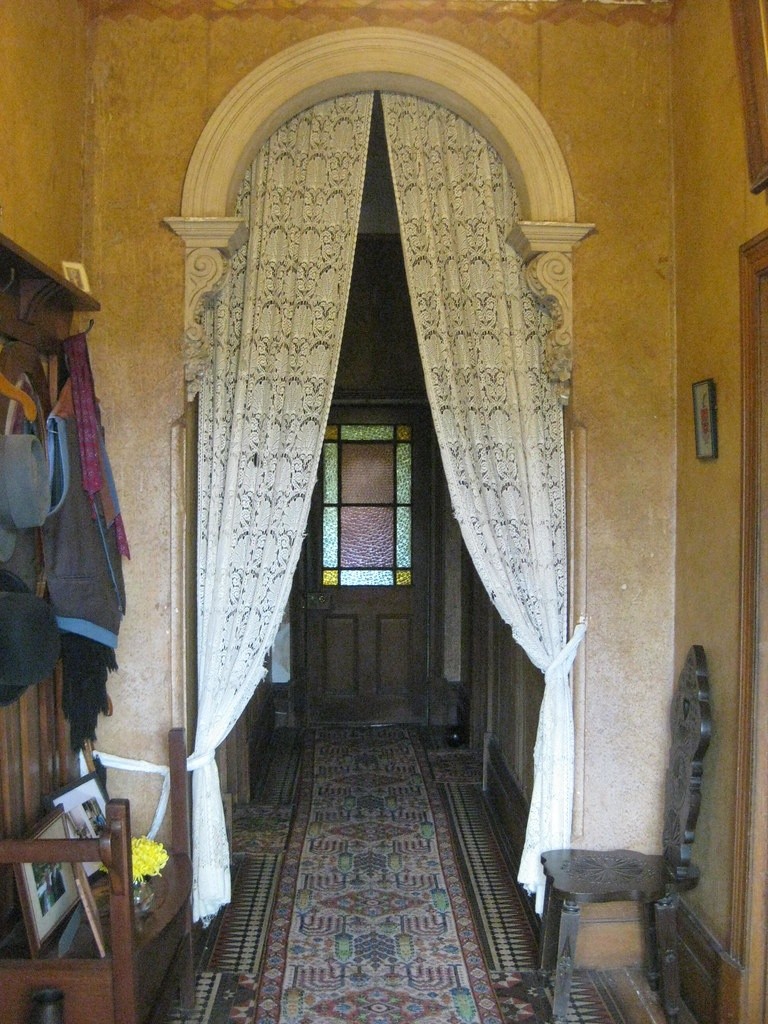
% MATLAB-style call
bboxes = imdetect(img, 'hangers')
[44,351,71,434]
[0,369,37,424]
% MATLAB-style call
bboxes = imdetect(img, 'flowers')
[98,833,170,884]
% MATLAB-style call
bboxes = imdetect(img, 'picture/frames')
[692,378,718,460]
[76,861,105,959]
[42,771,110,885]
[12,803,82,961]
[62,261,92,294]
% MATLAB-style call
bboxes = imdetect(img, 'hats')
[0,567,61,707]
[0,435,51,562]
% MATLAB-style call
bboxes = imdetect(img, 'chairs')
[535,645,713,1024]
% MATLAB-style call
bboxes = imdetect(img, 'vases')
[133,878,155,915]
